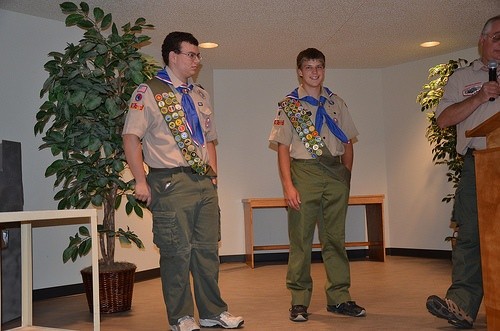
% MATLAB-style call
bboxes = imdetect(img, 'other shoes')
[288,305,308,321]
[326,301,367,317]
[426,294,475,328]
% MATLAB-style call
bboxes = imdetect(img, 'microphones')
[488,60,498,100]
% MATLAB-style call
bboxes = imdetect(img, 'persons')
[270,48,366,320]
[426,15,500,329]
[121,32,245,331]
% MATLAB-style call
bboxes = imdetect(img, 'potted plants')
[414,57,469,246]
[33,2,162,315]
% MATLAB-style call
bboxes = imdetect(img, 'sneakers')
[199,311,244,328]
[168,315,200,331]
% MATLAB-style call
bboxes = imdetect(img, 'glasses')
[481,32,500,42]
[177,51,204,61]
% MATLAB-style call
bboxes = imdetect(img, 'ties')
[284,87,350,144]
[157,69,207,149]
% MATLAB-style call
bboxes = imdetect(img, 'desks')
[241,194,387,269]
[0,209,103,331]
